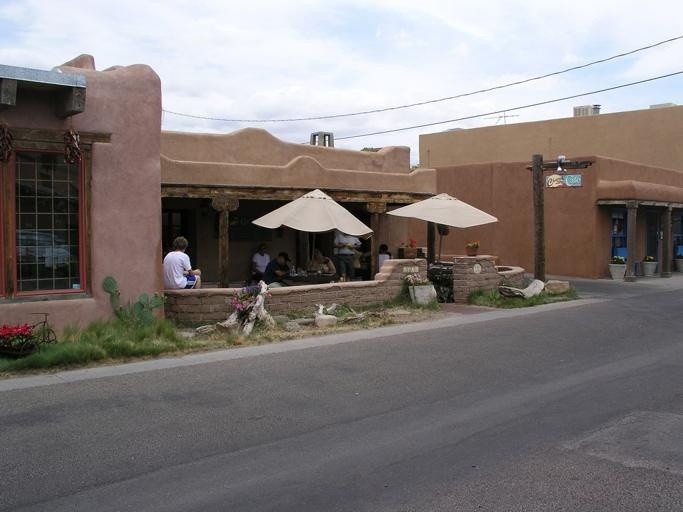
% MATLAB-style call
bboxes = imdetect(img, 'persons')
[333,234,361,280]
[305,246,336,275]
[252,243,291,288]
[163,237,201,290]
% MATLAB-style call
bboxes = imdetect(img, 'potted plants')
[464,238,479,256]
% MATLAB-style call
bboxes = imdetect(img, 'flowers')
[611,255,625,264]
[643,255,655,261]
[227,282,272,313]
[403,271,433,286]
[0,318,35,353]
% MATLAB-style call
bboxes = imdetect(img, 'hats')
[279,253,291,262]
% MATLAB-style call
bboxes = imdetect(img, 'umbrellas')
[386,193,497,260]
[252,189,374,260]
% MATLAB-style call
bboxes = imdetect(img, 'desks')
[282,270,337,285]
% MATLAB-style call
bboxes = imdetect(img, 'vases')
[608,263,628,280]
[641,261,660,277]
